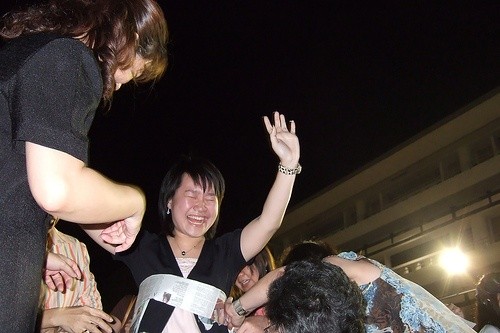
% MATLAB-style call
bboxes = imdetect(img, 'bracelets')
[278,164,302,174]
[232,299,249,316]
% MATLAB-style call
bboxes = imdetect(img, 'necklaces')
[173,235,203,255]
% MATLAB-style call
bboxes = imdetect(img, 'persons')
[0,0,170,333]
[38,111,500,333]
